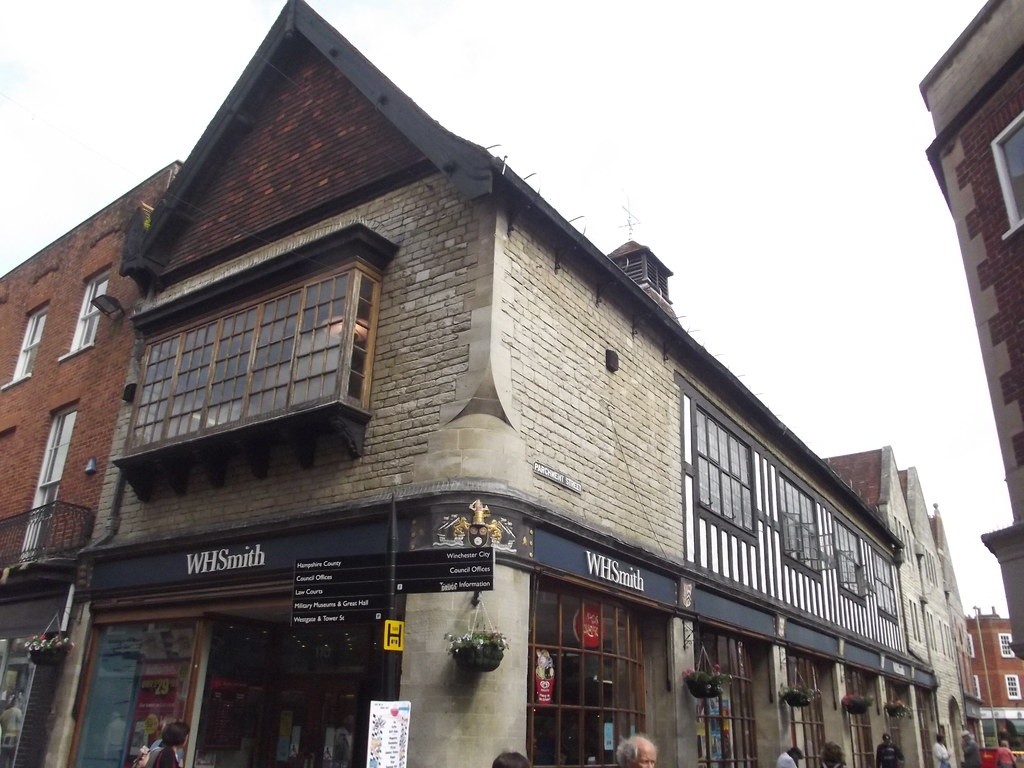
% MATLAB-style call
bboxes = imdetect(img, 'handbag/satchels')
[940,757,952,768]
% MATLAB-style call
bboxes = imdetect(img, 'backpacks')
[132,745,167,768]
[882,744,896,768]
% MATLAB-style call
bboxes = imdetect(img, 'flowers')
[841,690,873,708]
[884,701,913,719]
[444,627,511,657]
[684,662,734,698]
[24,632,74,658]
[779,683,817,703]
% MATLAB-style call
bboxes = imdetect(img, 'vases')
[457,646,503,672]
[687,680,724,698]
[30,648,66,664]
[848,705,868,714]
[886,709,897,717]
[786,695,810,708]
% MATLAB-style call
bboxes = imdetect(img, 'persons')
[776,730,1017,768]
[616,733,658,768]
[100,711,127,767]
[140,721,190,768]
[0,696,25,768]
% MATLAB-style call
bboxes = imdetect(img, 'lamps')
[321,314,368,342]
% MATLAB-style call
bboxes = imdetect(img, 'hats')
[960,730,969,736]
[882,733,890,739]
